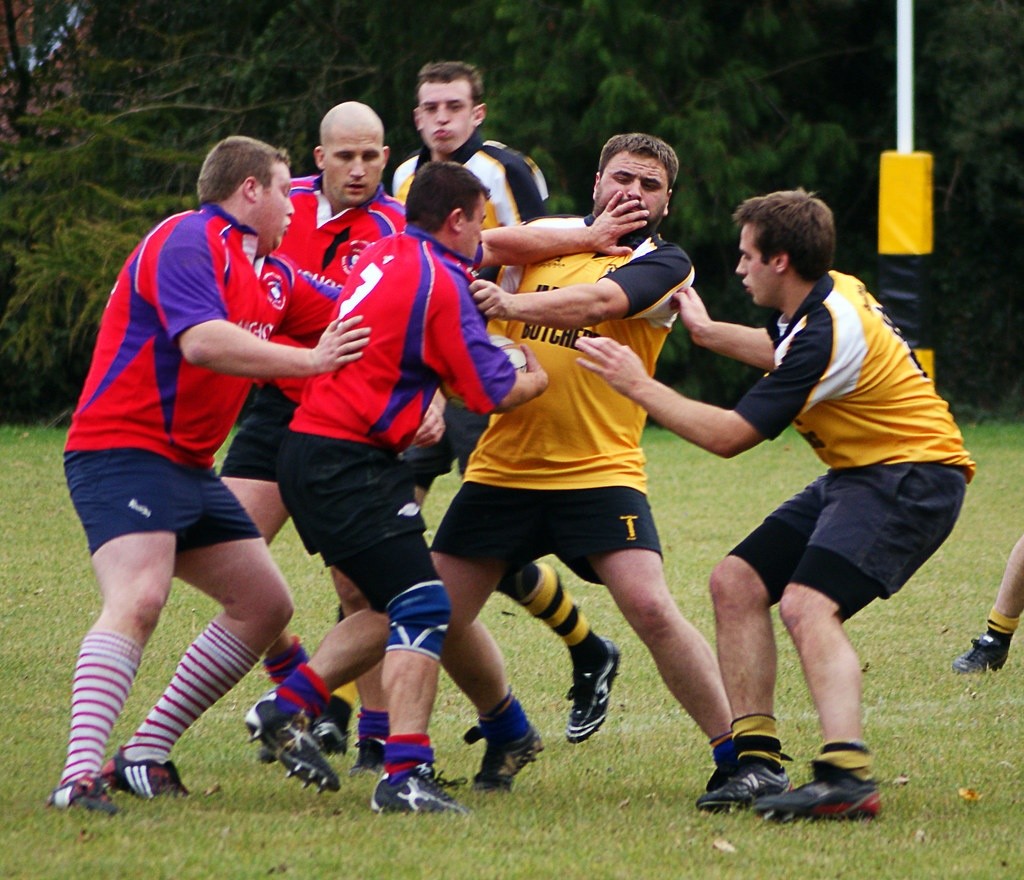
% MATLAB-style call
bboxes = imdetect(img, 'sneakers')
[348,736,387,778]
[243,683,341,793]
[370,770,474,819]
[693,763,795,815]
[565,637,622,745]
[752,758,881,822]
[45,778,117,816]
[951,633,1010,674]
[100,746,189,800]
[460,725,545,796]
[256,721,352,764]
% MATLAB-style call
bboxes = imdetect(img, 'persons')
[217,59,794,811]
[952,535,1024,675]
[47,136,372,817]
[575,186,974,821]
